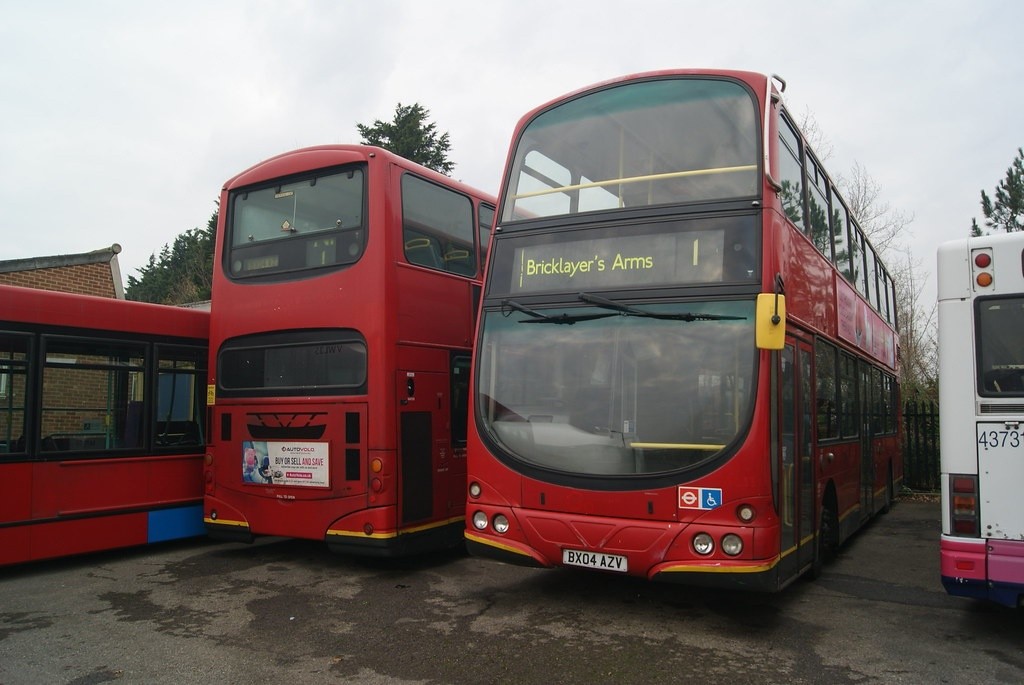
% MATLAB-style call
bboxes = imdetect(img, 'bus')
[0,283,212,569]
[199,141,747,563]
[461,65,907,613]
[937,227,1024,617]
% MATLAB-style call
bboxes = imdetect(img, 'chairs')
[403,239,478,280]
[0,400,152,454]
